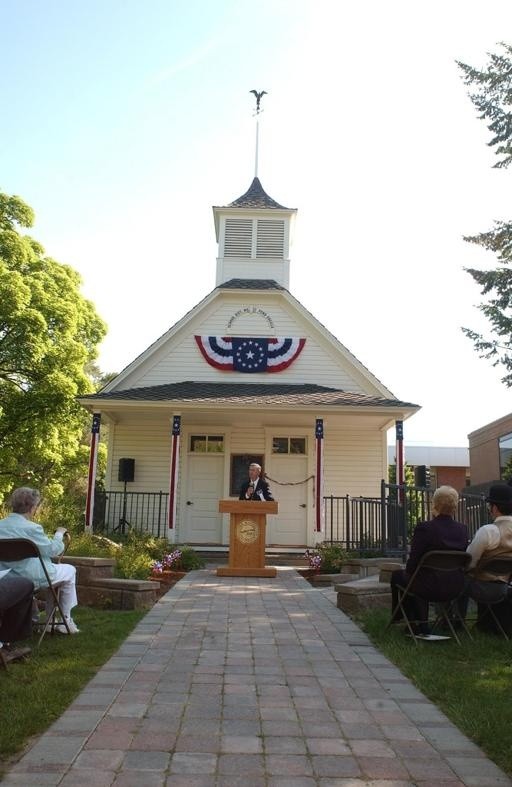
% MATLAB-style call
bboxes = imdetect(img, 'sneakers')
[0,645,32,665]
[39,616,81,634]
[404,623,429,636]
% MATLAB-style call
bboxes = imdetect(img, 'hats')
[484,483,511,504]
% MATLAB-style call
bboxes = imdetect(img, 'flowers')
[310,556,322,570]
[150,550,185,578]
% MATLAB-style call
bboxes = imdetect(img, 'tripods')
[113,481,134,534]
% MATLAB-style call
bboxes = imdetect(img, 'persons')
[0,487,81,635]
[239,462,274,502]
[391,485,468,636]
[0,577,35,664]
[439,485,512,626]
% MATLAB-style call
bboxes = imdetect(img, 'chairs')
[0,537,71,648]
[385,549,512,649]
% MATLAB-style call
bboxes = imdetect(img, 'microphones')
[250,482,254,495]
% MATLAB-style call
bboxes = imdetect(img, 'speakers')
[118,458,135,481]
